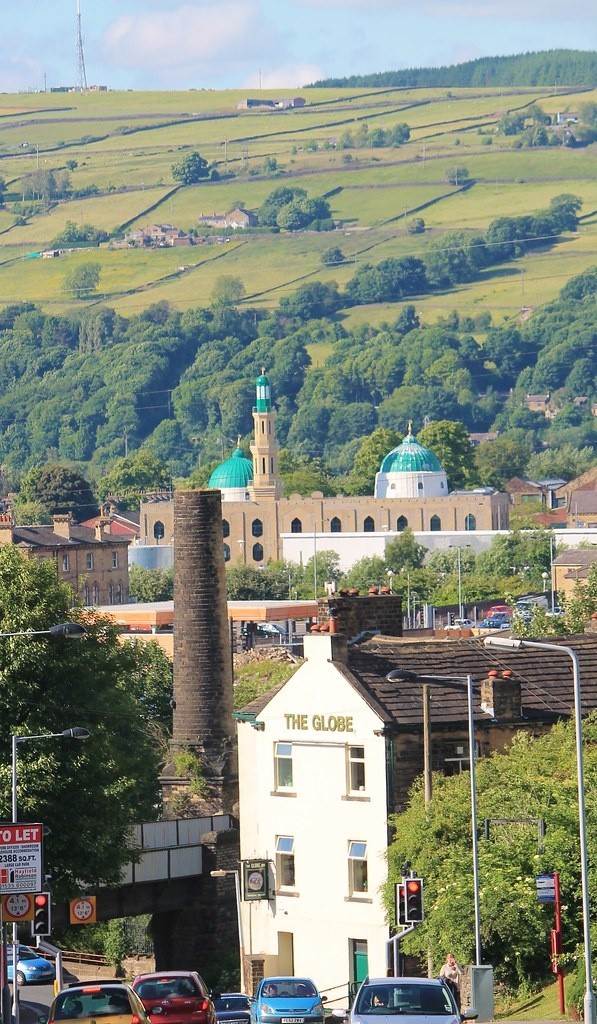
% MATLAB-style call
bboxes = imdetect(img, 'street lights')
[11,726,92,1024]
[541,572,549,590]
[386,570,395,596]
[482,636,597,1024]
[209,868,249,994]
[530,536,555,613]
[447,544,471,620]
[313,518,331,605]
[384,668,495,1022]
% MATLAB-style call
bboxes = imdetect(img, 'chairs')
[108,996,123,1005]
[141,984,157,998]
[419,991,445,1010]
[178,983,191,993]
[67,1001,83,1016]
[296,985,307,995]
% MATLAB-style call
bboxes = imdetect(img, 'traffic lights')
[30,891,51,937]
[404,877,425,923]
[394,883,414,928]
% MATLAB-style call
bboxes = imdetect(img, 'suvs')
[207,988,251,1024]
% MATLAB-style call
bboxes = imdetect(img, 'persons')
[374,989,389,1008]
[439,953,464,1013]
[169,981,188,994]
[266,984,278,997]
[109,996,125,1008]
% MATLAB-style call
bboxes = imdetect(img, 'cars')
[131,966,217,1024]
[5,944,56,986]
[249,977,328,1024]
[243,622,295,638]
[331,976,479,1024]
[443,595,567,631]
[37,976,163,1024]
